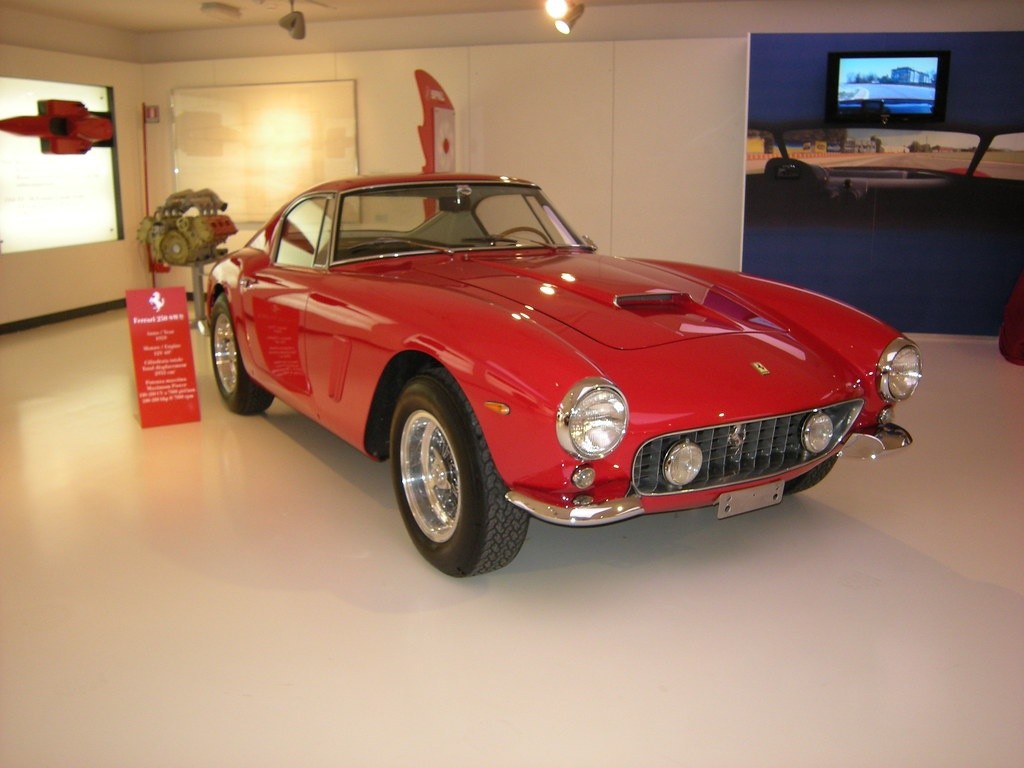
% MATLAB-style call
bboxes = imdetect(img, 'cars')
[203,172,924,578]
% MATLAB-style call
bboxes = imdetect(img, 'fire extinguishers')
[145,222,170,274]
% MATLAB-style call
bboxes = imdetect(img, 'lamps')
[543,0,584,34]
[279,0,305,38]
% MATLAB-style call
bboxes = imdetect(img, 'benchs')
[287,236,451,261]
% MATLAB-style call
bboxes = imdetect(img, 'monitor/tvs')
[824,48,950,123]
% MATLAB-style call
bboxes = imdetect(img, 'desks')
[169,257,216,328]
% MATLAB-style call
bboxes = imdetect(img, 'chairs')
[765,158,832,201]
